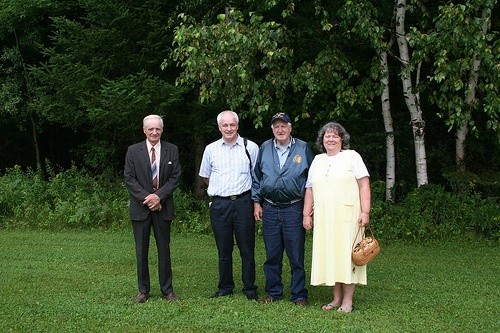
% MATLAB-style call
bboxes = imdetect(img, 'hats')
[270,112,292,124]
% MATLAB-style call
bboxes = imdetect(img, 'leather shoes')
[137,292,149,303]
[166,292,177,301]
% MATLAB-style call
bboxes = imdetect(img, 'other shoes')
[211,288,234,299]
[246,292,257,302]
[258,295,283,304]
[295,298,307,308]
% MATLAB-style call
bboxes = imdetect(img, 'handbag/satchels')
[351,226,381,266]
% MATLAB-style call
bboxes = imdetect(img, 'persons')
[198,111,259,299]
[124,115,182,303]
[303,122,371,313]
[252,113,314,305]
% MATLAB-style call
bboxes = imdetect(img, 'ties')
[150,148,158,191]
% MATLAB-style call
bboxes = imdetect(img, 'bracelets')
[362,210,370,214]
[303,215,312,217]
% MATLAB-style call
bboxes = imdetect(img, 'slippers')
[337,304,354,314]
[321,301,342,310]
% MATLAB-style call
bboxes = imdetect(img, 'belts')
[213,189,251,200]
[265,200,298,207]
